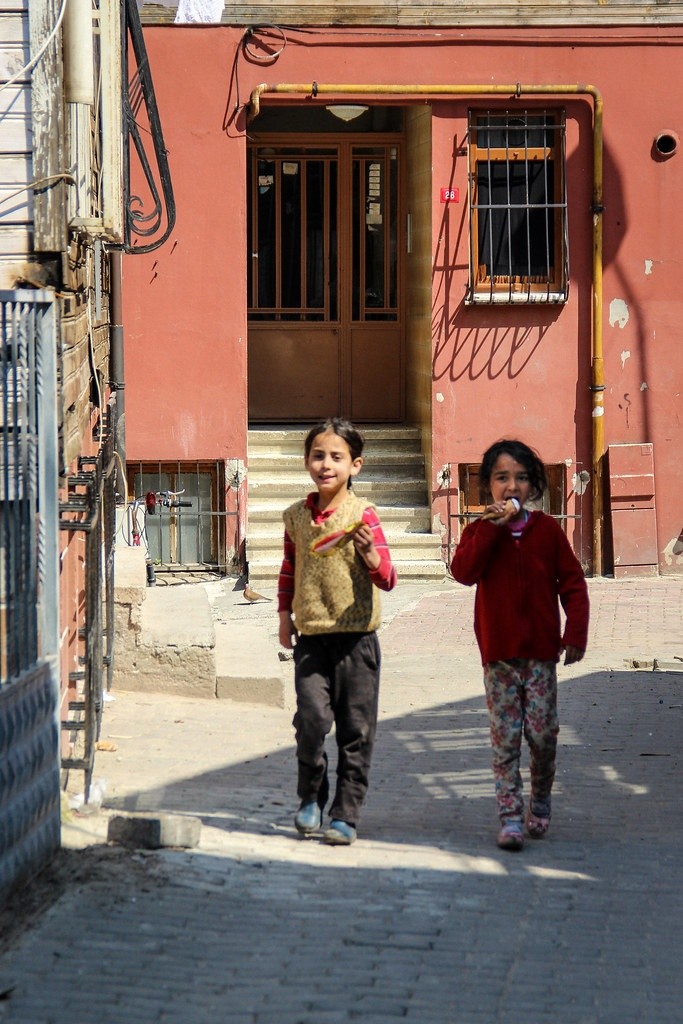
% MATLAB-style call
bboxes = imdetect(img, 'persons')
[450,437,590,849]
[277,417,398,846]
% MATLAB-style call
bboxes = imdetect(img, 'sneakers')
[496,824,523,848]
[324,817,357,845]
[527,806,552,835]
[295,801,322,833]
